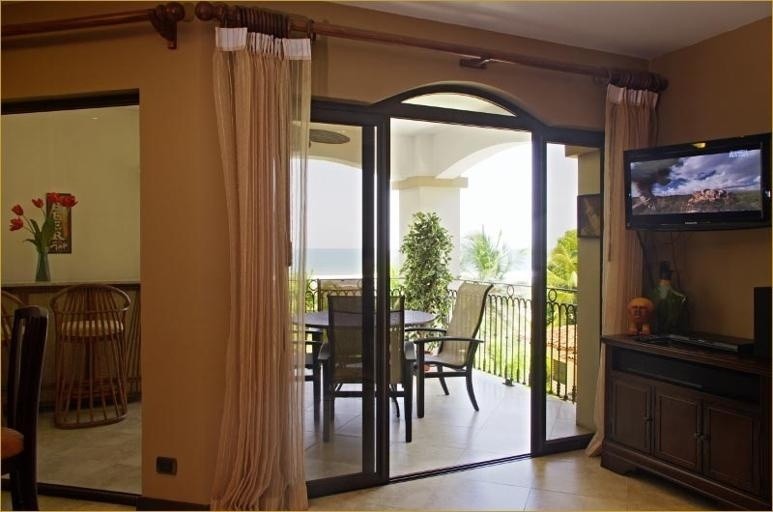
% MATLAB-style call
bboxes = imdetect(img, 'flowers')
[8,192,78,280]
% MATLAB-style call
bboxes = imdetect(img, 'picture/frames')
[577,193,601,238]
[45,193,72,254]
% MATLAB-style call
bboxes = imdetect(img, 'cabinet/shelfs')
[601,331,771,510]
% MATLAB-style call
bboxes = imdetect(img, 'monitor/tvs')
[624,132,772,231]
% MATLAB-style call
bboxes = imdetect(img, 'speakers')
[752,286,772,359]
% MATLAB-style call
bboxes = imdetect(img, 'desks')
[1,280,140,413]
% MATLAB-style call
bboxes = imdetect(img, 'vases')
[35,246,51,282]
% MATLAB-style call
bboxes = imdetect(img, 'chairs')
[289,277,493,443]
[1,290,27,428]
[49,282,132,430]
[0,305,49,511]
